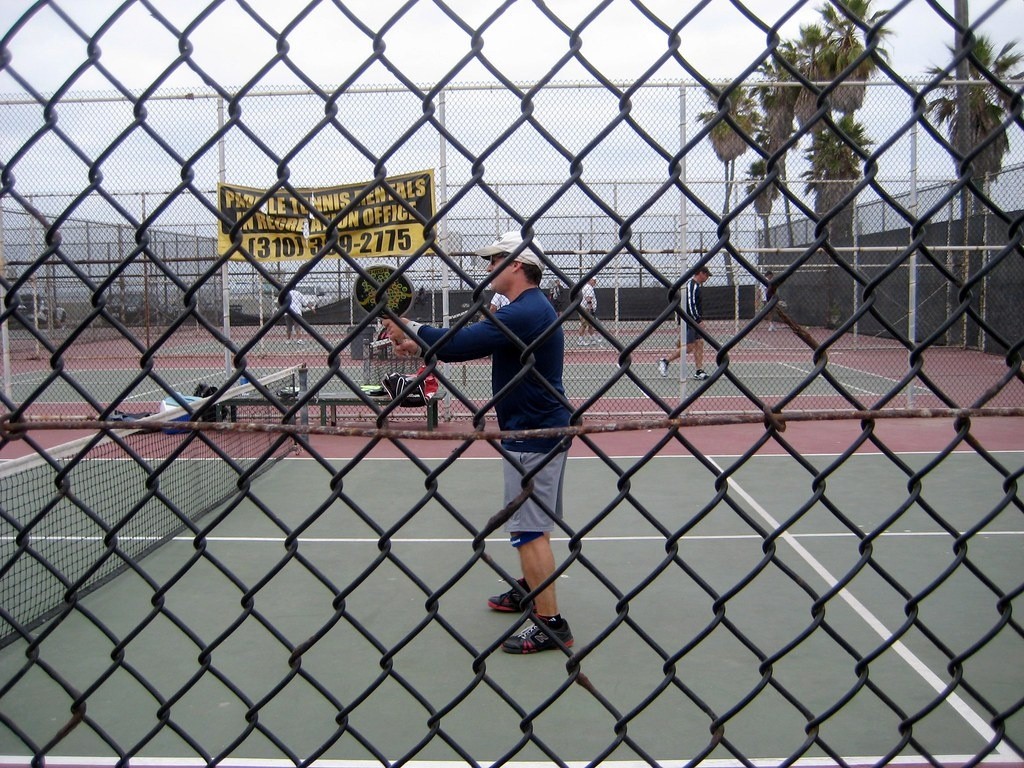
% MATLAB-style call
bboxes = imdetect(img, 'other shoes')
[578,338,590,345]
[285,340,291,344]
[297,340,305,344]
[591,336,603,345]
[768,325,777,332]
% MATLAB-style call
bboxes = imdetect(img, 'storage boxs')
[162,397,204,435]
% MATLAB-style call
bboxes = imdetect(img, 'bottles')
[239,375,249,397]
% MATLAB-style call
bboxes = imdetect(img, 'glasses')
[489,253,507,266]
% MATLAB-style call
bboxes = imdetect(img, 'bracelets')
[403,321,424,339]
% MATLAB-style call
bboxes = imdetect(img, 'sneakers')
[489,578,537,612]
[694,369,709,380]
[501,612,574,654]
[659,358,667,377]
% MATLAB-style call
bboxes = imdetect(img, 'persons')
[548,280,564,316]
[489,293,510,313]
[759,271,778,332]
[658,267,713,381]
[577,276,602,346]
[382,231,574,654]
[283,285,316,345]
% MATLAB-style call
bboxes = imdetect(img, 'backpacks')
[194,382,229,423]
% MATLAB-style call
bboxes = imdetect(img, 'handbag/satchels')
[558,292,568,303]
[382,372,439,408]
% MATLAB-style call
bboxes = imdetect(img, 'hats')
[475,232,546,273]
[695,266,713,276]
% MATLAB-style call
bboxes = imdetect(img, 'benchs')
[219,391,447,432]
